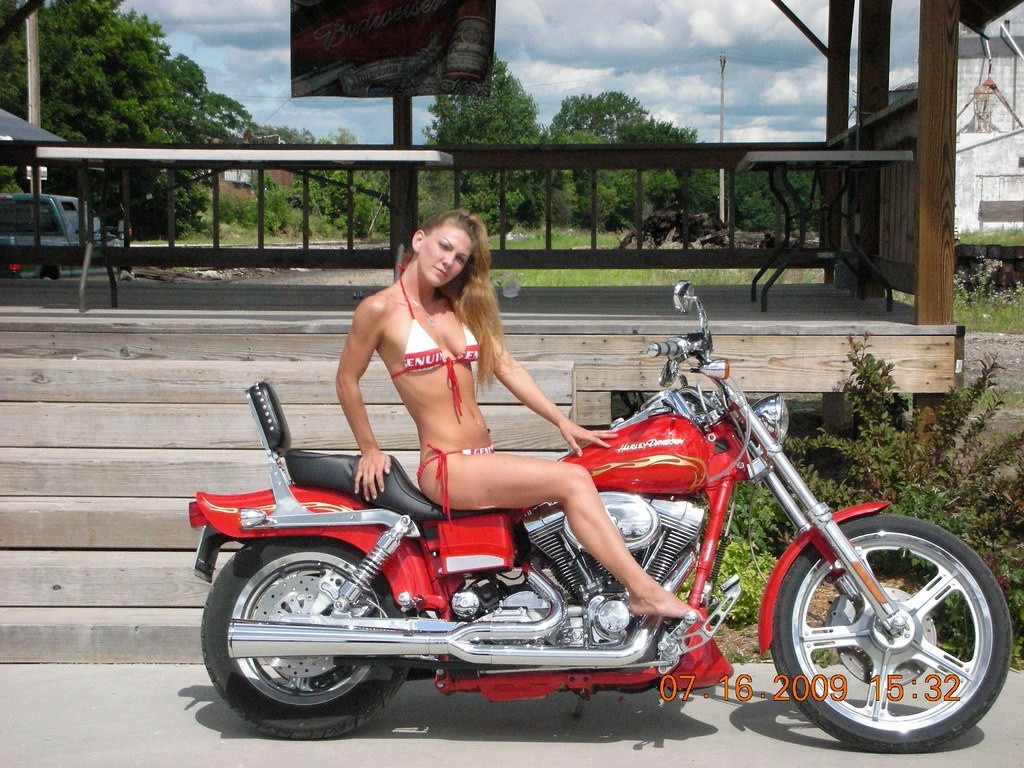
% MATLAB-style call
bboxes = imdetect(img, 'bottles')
[8,264,21,288]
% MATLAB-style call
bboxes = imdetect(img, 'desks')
[37,147,453,313]
[735,151,913,312]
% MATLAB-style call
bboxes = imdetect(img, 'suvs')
[0,194,125,276]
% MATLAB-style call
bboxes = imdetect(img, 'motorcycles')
[186,279,1014,755]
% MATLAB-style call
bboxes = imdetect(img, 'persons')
[336,206,709,624]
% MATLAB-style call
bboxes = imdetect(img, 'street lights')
[717,196,729,235]
